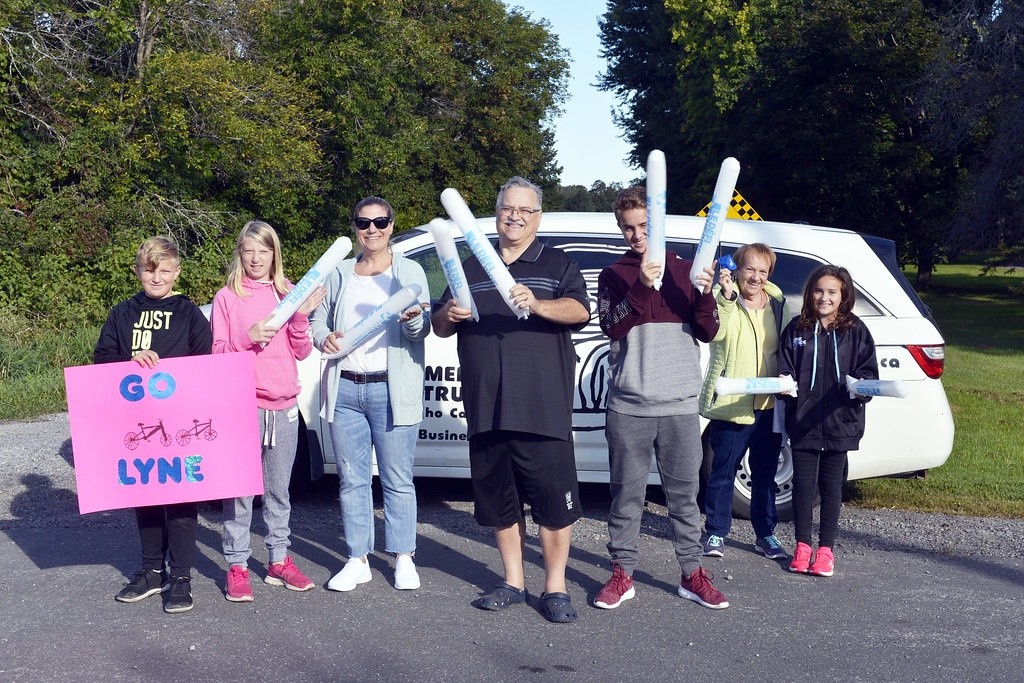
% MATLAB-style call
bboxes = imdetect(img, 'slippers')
[540,591,577,623]
[478,582,529,611]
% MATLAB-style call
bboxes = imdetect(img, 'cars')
[197,211,956,523]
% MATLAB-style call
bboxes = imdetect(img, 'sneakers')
[263,554,316,591]
[594,561,636,609]
[164,575,193,612]
[393,554,420,590]
[809,546,835,576]
[328,557,372,591]
[702,534,725,557]
[678,566,729,609]
[115,569,170,602]
[789,542,813,573]
[754,534,788,559]
[225,565,255,601]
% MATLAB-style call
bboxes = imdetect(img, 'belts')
[340,370,387,384]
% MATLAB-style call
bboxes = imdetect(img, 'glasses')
[354,216,393,230]
[497,204,540,216]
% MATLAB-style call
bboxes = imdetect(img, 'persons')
[93,233,212,613]
[432,175,591,623]
[773,265,880,576]
[311,196,432,591]
[697,244,788,559]
[209,219,327,601]
[592,187,729,609]
